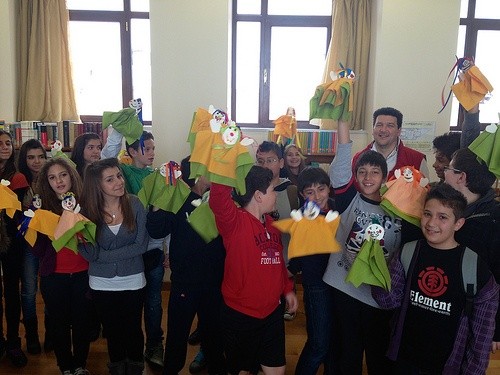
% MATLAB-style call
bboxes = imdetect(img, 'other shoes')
[74,367,87,375]
[62,370,72,375]
[188,328,201,344]
[284,307,296,322]
[145,338,163,366]
[109,360,125,375]
[128,358,144,375]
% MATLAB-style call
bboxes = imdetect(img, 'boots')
[22,320,41,354]
[43,320,52,351]
[5,338,27,368]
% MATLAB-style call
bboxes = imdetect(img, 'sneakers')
[189,347,209,373]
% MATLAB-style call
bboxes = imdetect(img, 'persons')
[432,57,480,181]
[281,143,306,321]
[370,181,498,375]
[440,149,500,355]
[144,153,225,375]
[60,157,151,375]
[251,139,301,263]
[314,71,428,375]
[68,132,110,342]
[97,99,172,363]
[286,166,339,375]
[209,123,297,375]
[0,130,28,367]
[351,103,430,203]
[25,158,94,375]
[12,138,57,354]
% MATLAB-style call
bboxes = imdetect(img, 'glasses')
[443,166,463,173]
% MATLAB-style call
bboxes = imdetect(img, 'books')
[265,126,336,157]
[0,114,112,152]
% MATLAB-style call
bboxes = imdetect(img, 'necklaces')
[105,205,121,218]
